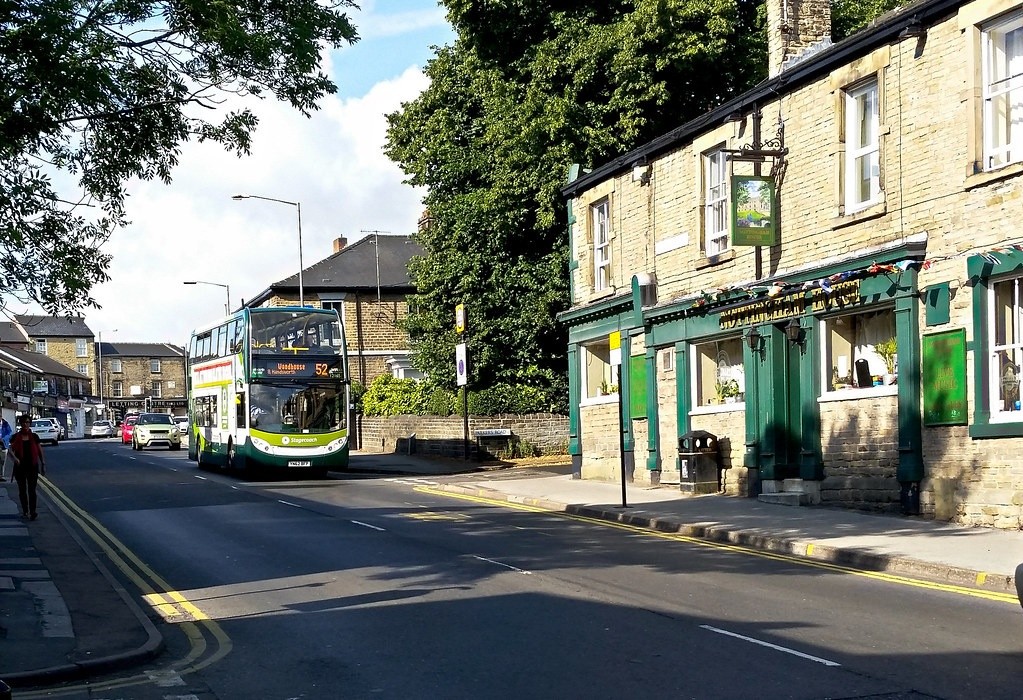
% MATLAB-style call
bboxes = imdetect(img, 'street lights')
[98,328,119,404]
[183,281,230,317]
[231,194,304,309]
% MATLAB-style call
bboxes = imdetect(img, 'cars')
[39,417,64,441]
[29,419,59,446]
[173,416,189,435]
[119,416,140,445]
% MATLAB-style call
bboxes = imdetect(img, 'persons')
[0,413,12,482]
[303,335,319,349]
[8,415,47,521]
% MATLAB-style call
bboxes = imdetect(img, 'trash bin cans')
[677,431,718,494]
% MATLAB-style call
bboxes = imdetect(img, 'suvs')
[131,413,182,452]
[91,420,118,438]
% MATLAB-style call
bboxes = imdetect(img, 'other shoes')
[29,512,38,520]
[23,510,28,516]
[1,476,7,481]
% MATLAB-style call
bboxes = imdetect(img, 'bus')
[187,305,352,479]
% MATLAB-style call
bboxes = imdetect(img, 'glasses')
[19,420,31,424]
